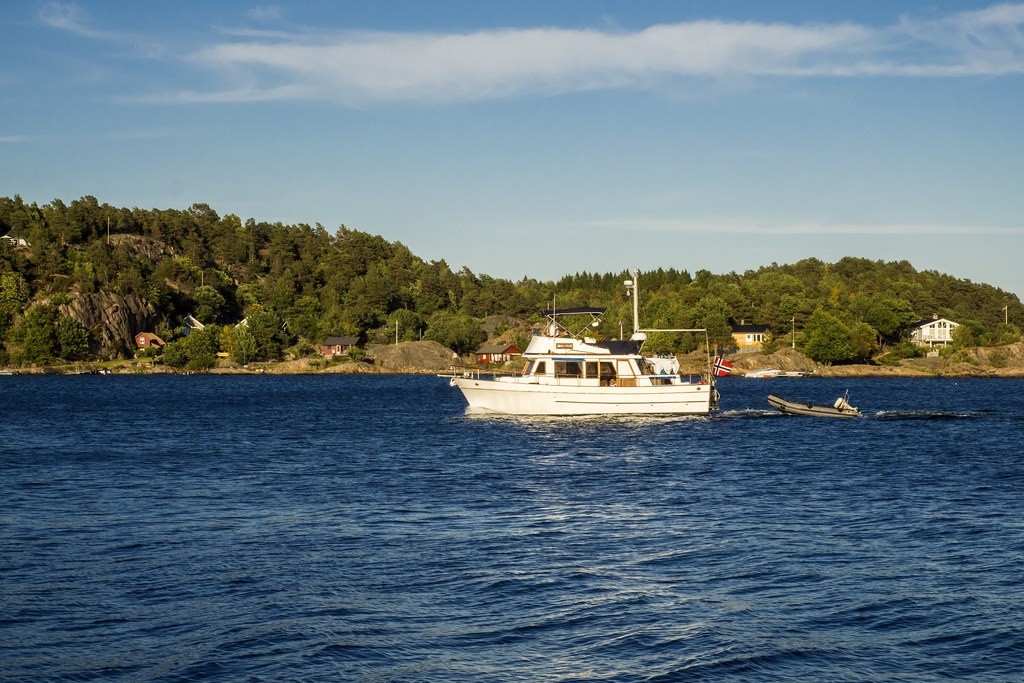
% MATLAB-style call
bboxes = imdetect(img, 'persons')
[600,375,617,386]
[562,329,570,338]
[710,386,721,407]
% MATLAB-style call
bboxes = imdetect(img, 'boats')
[437,270,727,420]
[766,394,871,421]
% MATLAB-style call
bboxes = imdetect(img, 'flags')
[712,355,733,377]
